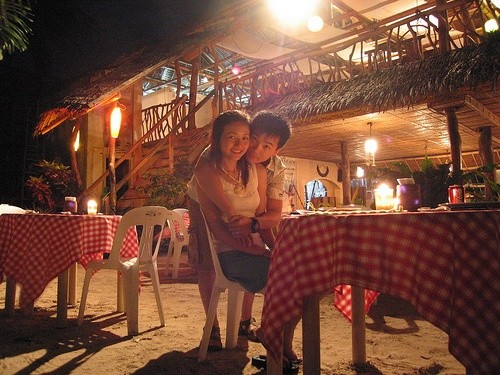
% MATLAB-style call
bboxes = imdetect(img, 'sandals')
[199,326,222,350]
[239,317,262,343]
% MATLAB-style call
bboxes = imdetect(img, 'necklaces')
[221,166,244,194]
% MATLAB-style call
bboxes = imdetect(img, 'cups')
[87,203,97,215]
[396,184,421,212]
[64,200,77,213]
[374,189,393,210]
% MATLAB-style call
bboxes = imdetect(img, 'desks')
[263,205,500,375]
[0,212,140,323]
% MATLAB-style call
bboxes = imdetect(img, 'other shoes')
[253,326,303,364]
[252,355,299,374]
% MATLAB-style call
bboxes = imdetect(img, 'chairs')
[77,205,259,365]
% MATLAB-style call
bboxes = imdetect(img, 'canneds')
[448,184,465,203]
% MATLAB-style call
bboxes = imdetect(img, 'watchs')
[251,217,260,233]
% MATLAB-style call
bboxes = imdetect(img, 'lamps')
[365,121,378,155]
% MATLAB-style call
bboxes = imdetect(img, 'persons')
[187,109,291,349]
[196,110,302,372]
